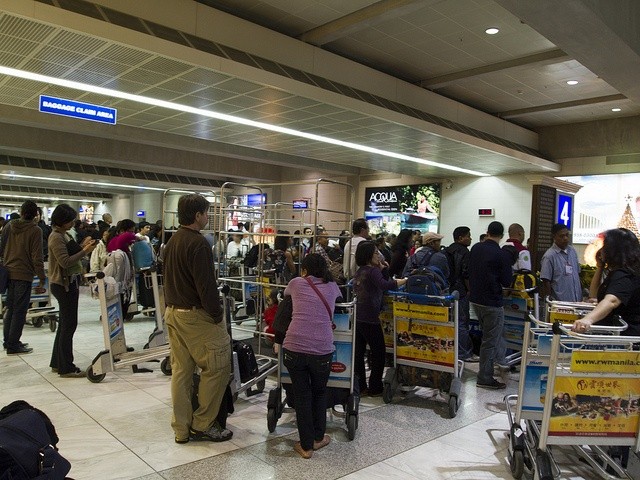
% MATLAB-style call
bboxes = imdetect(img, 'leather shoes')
[3,343,28,350]
[6,347,34,354]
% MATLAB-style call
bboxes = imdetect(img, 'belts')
[167,303,201,310]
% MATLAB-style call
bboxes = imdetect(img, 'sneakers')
[505,365,516,372]
[60,367,87,378]
[293,441,313,459]
[458,353,480,363]
[189,422,233,442]
[370,389,385,397]
[174,433,190,444]
[476,377,506,389]
[359,389,369,397]
[51,366,60,371]
[312,435,331,451]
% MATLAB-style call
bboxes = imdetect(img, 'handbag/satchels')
[282,371,360,410]
[241,243,270,268]
[231,339,259,383]
[325,246,343,265]
[191,373,234,430]
[67,240,83,256]
[0,263,10,295]
[132,238,157,270]
[0,400,72,480]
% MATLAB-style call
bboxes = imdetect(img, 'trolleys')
[503,312,628,479]
[380,284,464,417]
[266,297,360,441]
[524,321,640,480]
[88,258,141,322]
[0,262,59,332]
[545,294,597,322]
[494,286,545,366]
[192,286,278,405]
[84,262,172,382]
[139,263,169,349]
[217,257,257,326]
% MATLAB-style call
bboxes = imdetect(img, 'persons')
[243,243,271,300]
[414,229,423,245]
[1,201,47,356]
[402,233,451,294]
[237,222,244,235]
[284,253,344,459]
[540,222,582,319]
[239,222,256,258]
[308,230,342,281]
[382,234,397,263]
[159,193,233,444]
[211,230,224,263]
[388,228,411,279]
[409,193,436,215]
[382,232,388,238]
[596,263,604,267]
[342,219,389,330]
[339,230,351,250]
[524,238,530,249]
[352,241,407,397]
[313,225,324,243]
[468,221,517,388]
[35,207,51,257]
[479,233,488,243]
[224,233,243,259]
[274,228,298,283]
[301,227,312,257]
[46,201,96,379]
[409,230,416,245]
[499,223,532,308]
[571,228,639,350]
[410,240,421,256]
[378,236,388,259]
[76,214,159,323]
[439,226,481,363]
[376,234,382,239]
[291,230,306,256]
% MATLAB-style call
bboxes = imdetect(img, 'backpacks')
[509,268,537,309]
[439,244,462,289]
[405,249,450,306]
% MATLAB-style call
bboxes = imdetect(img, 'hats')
[423,232,444,245]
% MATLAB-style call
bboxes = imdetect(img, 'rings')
[580,325,585,328]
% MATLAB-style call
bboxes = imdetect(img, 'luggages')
[224,244,248,302]
